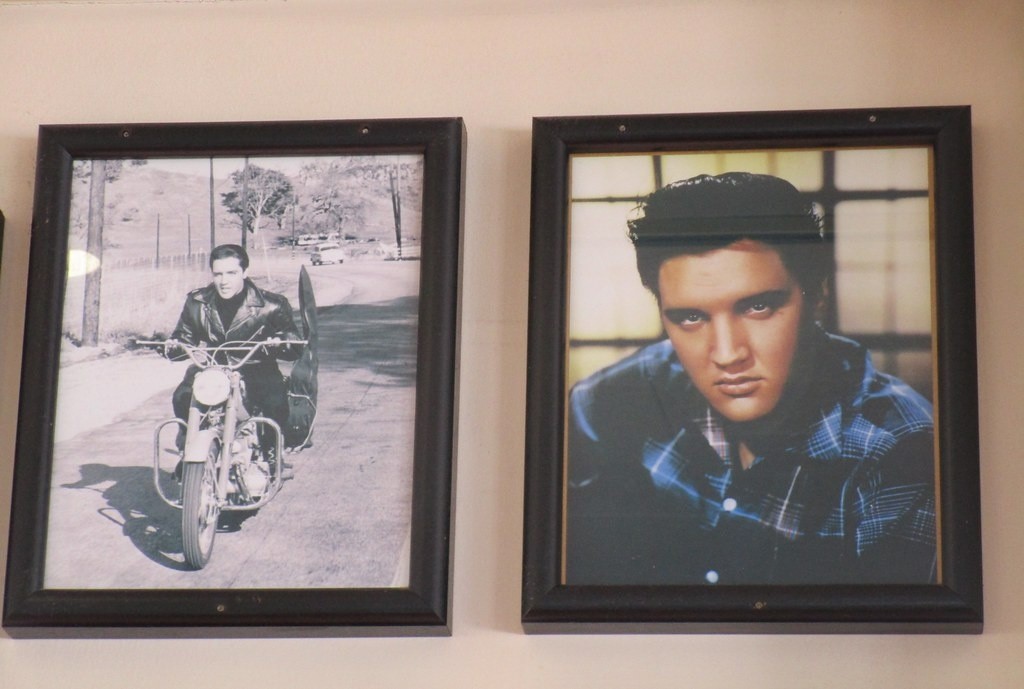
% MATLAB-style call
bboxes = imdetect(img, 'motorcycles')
[138,337,310,569]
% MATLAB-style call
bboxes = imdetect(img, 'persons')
[567,170,942,587]
[156,243,306,480]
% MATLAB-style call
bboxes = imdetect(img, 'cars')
[310,243,345,265]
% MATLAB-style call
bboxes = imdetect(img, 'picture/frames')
[0,117,469,628]
[521,103,986,625]
